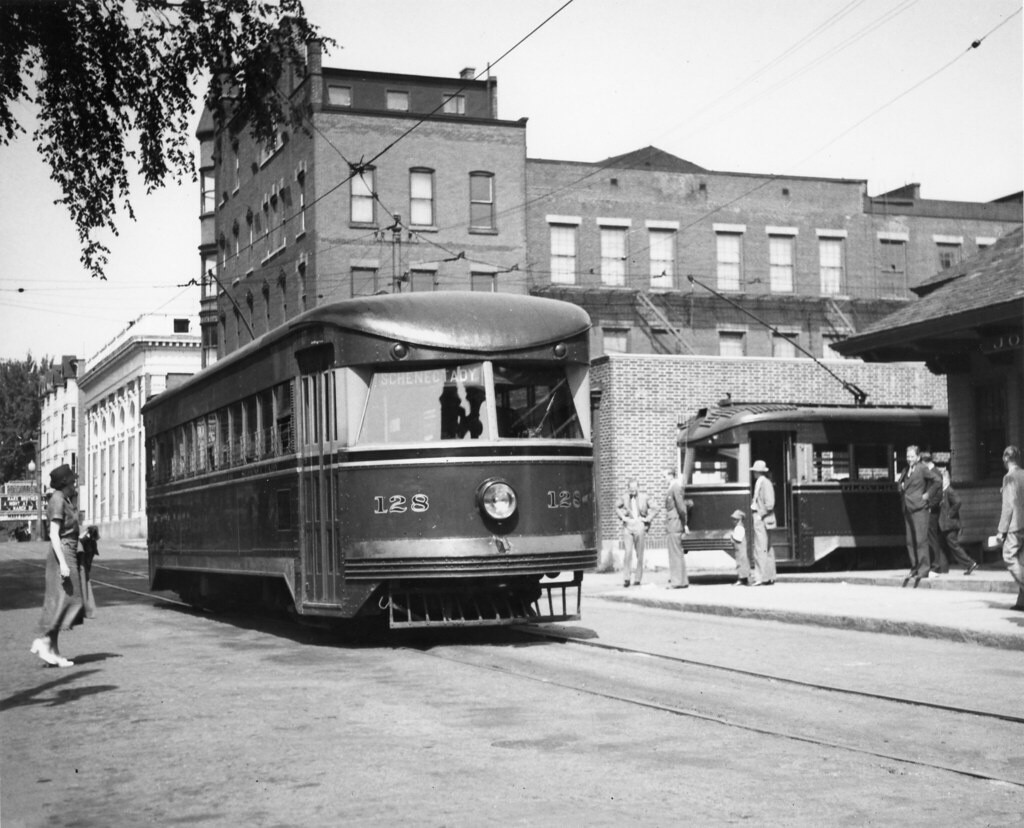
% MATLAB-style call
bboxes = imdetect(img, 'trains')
[677,397,949,577]
[140,293,600,629]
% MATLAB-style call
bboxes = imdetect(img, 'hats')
[730,509,746,521]
[749,460,769,472]
[50,463,80,489]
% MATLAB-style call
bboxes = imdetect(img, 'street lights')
[26,460,38,509]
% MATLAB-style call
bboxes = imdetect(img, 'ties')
[631,496,639,521]
[908,466,912,478]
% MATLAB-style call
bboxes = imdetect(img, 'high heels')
[30,637,59,664]
[49,646,74,668]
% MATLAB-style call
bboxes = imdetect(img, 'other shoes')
[964,561,979,575]
[732,578,747,587]
[1010,605,1024,610]
[623,581,630,587]
[905,570,917,580]
[752,580,775,586]
[911,572,928,580]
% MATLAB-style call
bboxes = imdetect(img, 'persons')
[996,445,1024,611]
[729,459,779,586]
[896,445,979,580]
[29,464,85,669]
[613,480,662,588]
[77,510,97,619]
[664,468,691,590]
